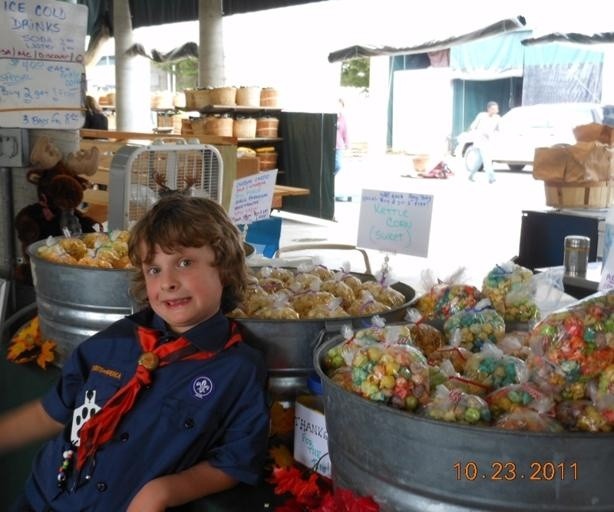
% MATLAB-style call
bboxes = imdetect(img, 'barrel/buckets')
[225,272,417,409]
[25,232,147,365]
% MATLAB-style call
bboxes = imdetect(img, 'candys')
[325,268,614,432]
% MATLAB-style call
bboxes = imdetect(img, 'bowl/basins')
[23,232,420,411]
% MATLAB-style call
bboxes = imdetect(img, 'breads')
[227,265,406,319]
[36,231,135,268]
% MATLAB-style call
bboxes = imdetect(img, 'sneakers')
[469,174,496,186]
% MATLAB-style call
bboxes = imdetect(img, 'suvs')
[452,101,614,182]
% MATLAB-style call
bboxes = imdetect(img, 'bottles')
[563,235,591,275]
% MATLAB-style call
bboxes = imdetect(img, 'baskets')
[544,180,613,209]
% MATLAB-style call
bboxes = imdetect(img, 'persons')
[83,97,109,190]
[0,193,273,511]
[460,99,506,185]
